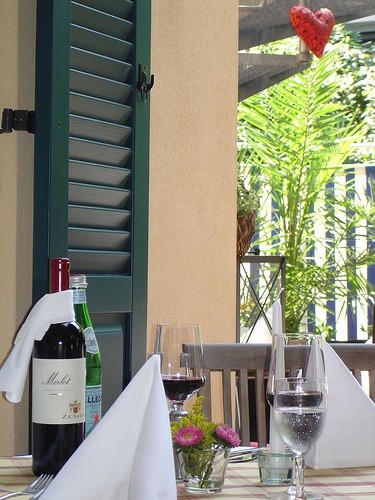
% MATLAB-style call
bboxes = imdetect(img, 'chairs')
[181,341,374,447]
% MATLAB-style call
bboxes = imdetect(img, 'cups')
[257,448,294,486]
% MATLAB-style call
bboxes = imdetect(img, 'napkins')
[0,289,76,404]
[38,353,177,500]
[301,338,375,470]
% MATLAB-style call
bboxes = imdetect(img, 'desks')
[0,446,375,500]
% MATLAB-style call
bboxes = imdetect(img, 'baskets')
[236,209,260,260]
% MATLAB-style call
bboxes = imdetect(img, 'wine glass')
[154,324,207,482]
[148,351,190,482]
[266,333,328,500]
[273,377,328,500]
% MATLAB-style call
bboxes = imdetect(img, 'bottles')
[30,257,103,479]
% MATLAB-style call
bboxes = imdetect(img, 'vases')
[175,444,230,495]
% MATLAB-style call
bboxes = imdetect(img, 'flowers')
[171,395,243,489]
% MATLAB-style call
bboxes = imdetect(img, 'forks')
[0,473,54,500]
